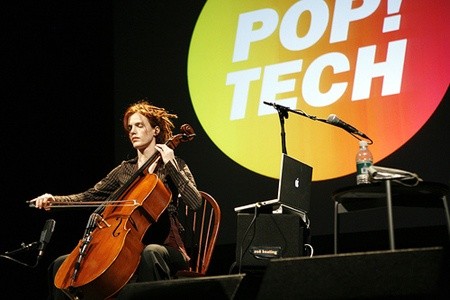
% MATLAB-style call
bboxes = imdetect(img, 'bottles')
[356,141,373,185]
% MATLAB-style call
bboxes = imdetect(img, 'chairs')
[176,191,221,280]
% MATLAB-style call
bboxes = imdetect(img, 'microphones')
[327,114,369,139]
[36,219,56,259]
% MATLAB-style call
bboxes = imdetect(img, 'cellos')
[53,123,196,300]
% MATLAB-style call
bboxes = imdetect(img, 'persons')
[30,100,202,300]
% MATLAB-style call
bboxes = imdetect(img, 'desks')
[331,181,450,254]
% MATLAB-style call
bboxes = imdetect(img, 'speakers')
[236,213,310,267]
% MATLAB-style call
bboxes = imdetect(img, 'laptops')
[234,153,313,213]
